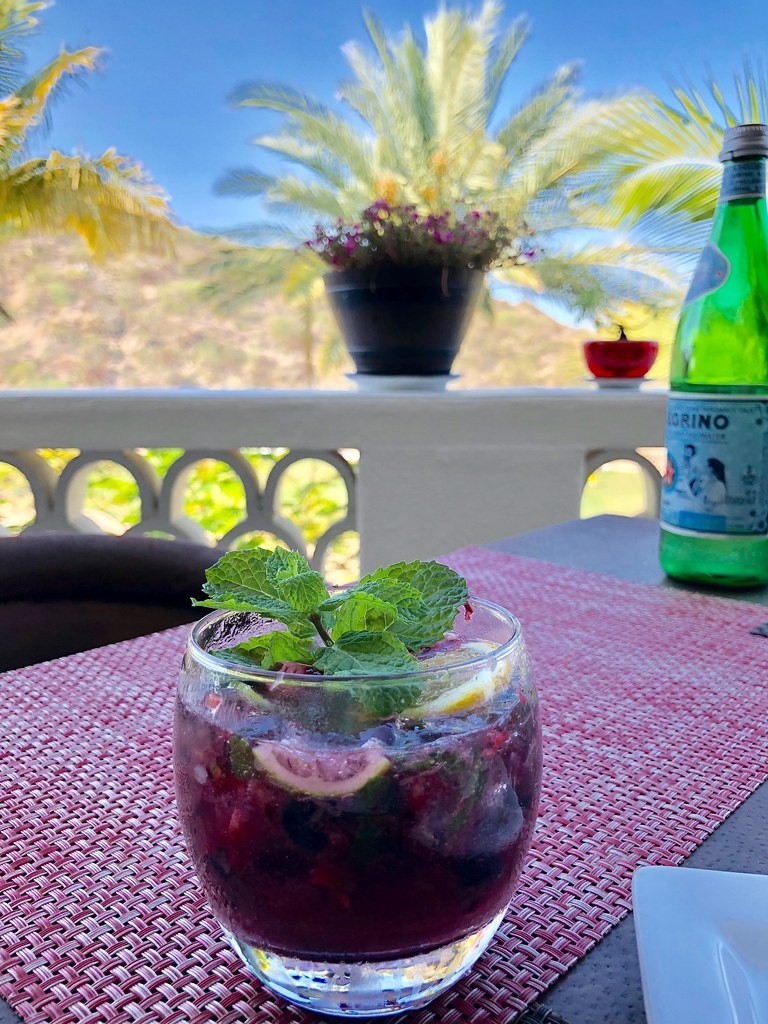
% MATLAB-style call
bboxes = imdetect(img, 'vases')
[318,257,485,389]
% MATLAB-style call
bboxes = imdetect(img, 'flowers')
[224,1,607,279]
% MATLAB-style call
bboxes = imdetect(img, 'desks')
[0,510,767,1024]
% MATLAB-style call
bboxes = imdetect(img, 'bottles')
[656,124,767,591]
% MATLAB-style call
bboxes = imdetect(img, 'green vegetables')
[187,543,470,723]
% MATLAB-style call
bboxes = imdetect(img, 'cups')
[173,594,544,1018]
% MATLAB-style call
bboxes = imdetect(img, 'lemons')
[394,640,509,722]
[249,742,392,799]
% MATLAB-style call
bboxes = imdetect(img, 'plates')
[633,865,768,1024]
